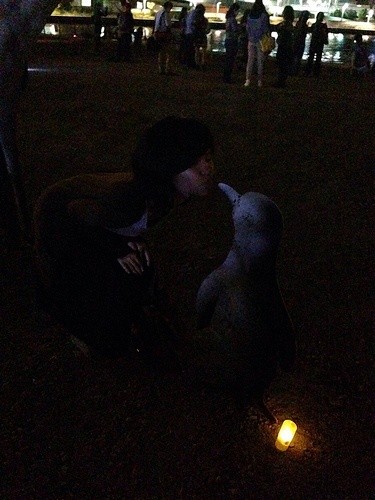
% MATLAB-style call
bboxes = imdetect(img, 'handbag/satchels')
[260,34,276,56]
[100,39,121,58]
[155,25,171,40]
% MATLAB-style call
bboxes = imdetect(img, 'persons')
[222,3,246,83]
[118,3,144,58]
[242,1,270,86]
[304,11,328,77]
[155,1,174,74]
[178,5,210,69]
[352,34,371,71]
[240,9,250,64]
[46,110,215,364]
[293,11,309,64]
[94,2,108,39]
[271,13,295,88]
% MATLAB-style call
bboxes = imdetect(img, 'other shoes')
[244,79,251,87]
[158,72,167,75]
[257,79,264,87]
[222,79,235,84]
[167,71,180,77]
